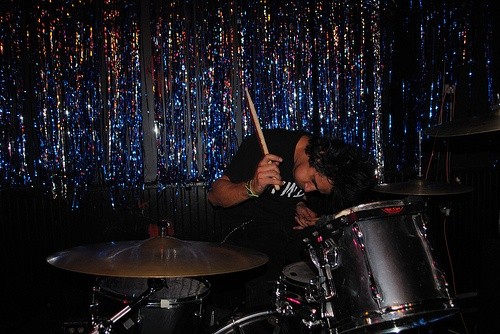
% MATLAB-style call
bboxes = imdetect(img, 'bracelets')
[244,180,259,199]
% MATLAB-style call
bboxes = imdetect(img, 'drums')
[267,259,331,334]
[90,276,213,334]
[301,197,461,334]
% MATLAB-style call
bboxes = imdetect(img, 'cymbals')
[425,108,500,137]
[212,309,273,334]
[46,218,272,281]
[371,172,476,197]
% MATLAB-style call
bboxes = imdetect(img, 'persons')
[207,129,373,307]
